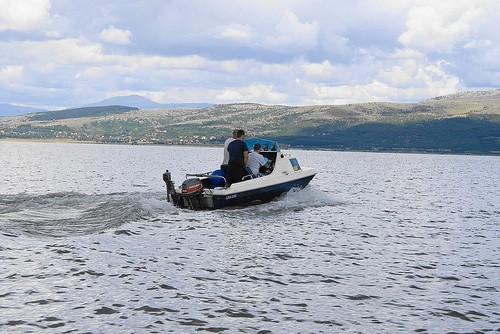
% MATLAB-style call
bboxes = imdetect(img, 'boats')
[163,137,319,209]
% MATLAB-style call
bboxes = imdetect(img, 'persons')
[226,130,249,186]
[246,143,272,179]
[221,129,239,175]
